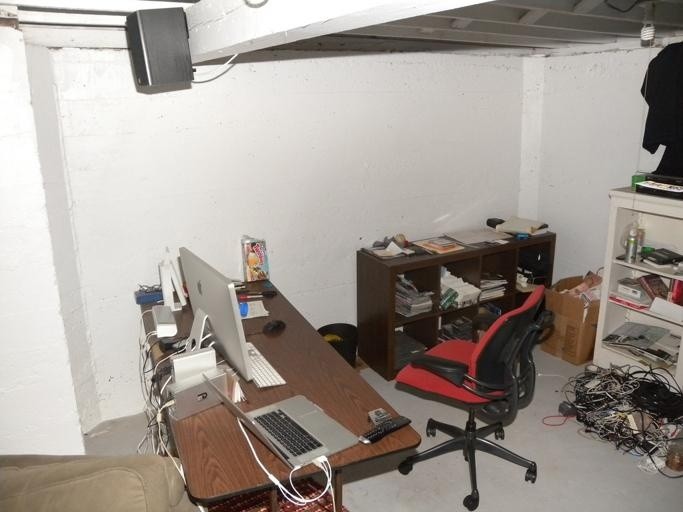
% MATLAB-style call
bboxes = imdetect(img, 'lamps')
[640,2,655,40]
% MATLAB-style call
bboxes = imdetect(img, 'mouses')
[263,318,284,338]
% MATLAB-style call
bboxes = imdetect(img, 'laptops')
[202,373,359,472]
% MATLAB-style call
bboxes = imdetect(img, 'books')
[608,271,671,313]
[398,232,509,317]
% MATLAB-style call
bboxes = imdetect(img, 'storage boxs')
[540,272,602,365]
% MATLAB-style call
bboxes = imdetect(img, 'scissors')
[248,291,276,298]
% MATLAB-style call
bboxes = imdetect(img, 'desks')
[141,279,421,512]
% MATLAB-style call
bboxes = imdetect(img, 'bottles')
[623,226,645,265]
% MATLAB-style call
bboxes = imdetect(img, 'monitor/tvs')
[179,243,254,382]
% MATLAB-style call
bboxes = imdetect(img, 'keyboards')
[245,340,286,388]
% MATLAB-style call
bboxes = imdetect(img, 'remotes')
[359,415,412,444]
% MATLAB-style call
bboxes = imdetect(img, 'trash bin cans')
[317,323,358,370]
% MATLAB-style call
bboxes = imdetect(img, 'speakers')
[126,8,195,94]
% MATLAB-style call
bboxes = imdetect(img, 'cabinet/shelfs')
[357,230,556,381]
[593,187,683,392]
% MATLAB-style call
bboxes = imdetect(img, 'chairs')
[398,285,545,512]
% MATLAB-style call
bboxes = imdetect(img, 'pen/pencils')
[240,295,263,299]
